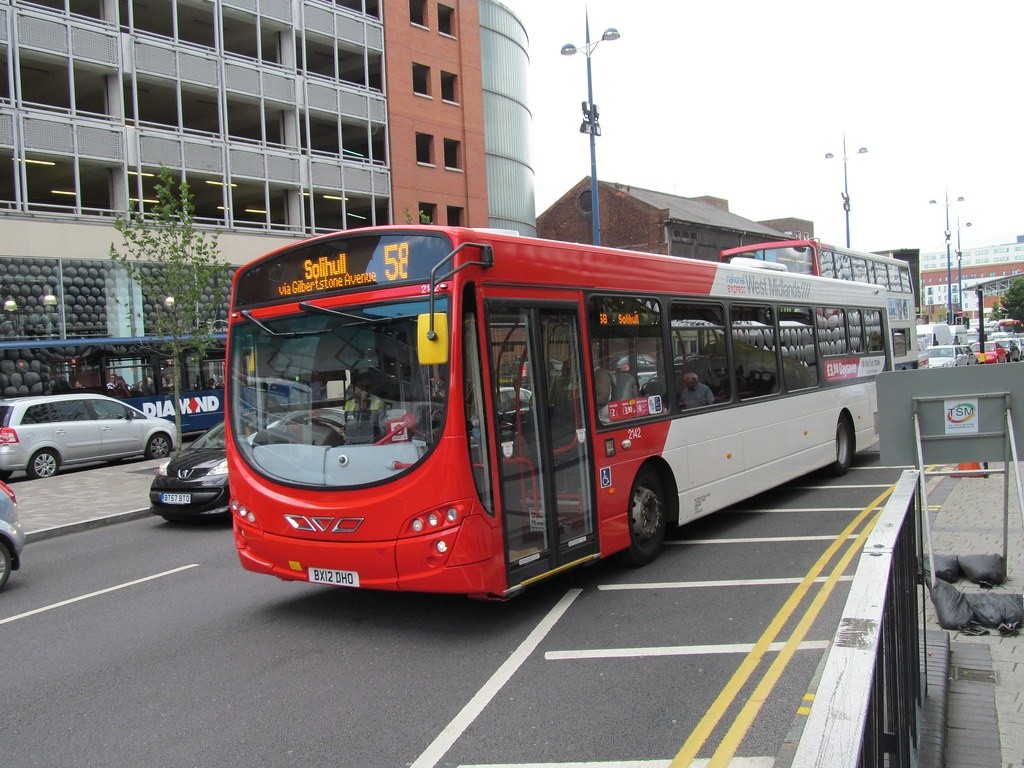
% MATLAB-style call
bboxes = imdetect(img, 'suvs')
[0,393,177,480]
[494,357,565,389]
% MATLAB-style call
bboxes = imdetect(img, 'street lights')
[931,180,971,321]
[43,295,58,339]
[825,137,869,251]
[165,297,176,335]
[4,297,21,340]
[561,5,622,244]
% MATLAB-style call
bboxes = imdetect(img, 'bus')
[0,335,328,432]
[224,226,920,602]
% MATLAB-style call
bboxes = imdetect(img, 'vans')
[917,324,967,347]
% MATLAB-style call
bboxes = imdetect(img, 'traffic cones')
[949,462,986,478]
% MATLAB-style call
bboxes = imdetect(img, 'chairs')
[58,375,148,398]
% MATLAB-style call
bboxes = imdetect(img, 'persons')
[338,373,387,424]
[679,371,715,407]
[75,372,224,397]
[592,365,615,412]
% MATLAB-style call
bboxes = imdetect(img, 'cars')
[916,334,1024,369]
[496,386,533,416]
[0,479,26,591]
[611,352,658,390]
[150,407,386,527]
[674,356,697,372]
[967,318,1024,340]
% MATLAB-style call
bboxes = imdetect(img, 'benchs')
[641,340,745,400]
